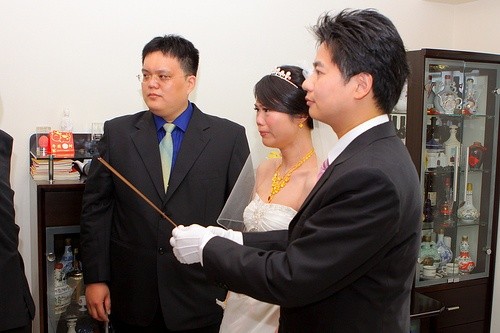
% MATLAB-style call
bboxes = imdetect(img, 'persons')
[170,8,425,333]
[217,66,341,333]
[0,131,35,333]
[79,35,255,333]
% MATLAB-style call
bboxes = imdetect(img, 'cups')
[419,265,436,277]
[442,263,459,274]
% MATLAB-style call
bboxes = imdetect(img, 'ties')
[317,159,329,182]
[159,123,175,195]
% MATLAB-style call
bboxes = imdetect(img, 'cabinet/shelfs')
[393,49,500,333]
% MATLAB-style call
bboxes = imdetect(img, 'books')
[32,159,79,181]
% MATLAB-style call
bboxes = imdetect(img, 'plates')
[420,272,443,279]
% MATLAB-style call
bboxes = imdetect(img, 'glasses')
[137,75,188,84]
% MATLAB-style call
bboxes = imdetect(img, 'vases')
[456,191,479,222]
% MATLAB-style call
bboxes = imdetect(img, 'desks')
[28,178,110,333]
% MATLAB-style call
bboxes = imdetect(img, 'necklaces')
[268,149,314,203]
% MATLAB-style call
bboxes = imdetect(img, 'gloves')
[170,223,244,267]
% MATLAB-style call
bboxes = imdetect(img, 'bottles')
[53,238,84,333]
[60,110,72,132]
[424,173,480,222]
[463,78,481,113]
[468,142,484,169]
[426,117,460,170]
[419,229,476,274]
[434,75,458,114]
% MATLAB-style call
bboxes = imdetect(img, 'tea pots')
[437,93,463,113]
